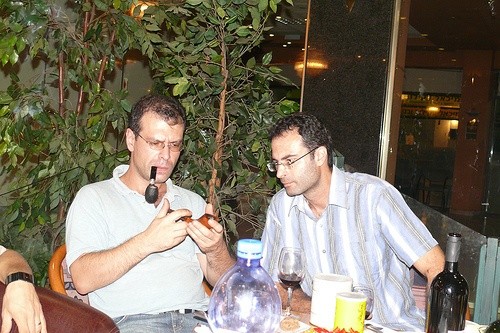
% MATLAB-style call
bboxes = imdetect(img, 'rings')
[35,322,41,325]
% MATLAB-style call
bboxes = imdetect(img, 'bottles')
[208,238,281,333]
[427,233,470,333]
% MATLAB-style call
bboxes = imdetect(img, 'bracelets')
[4,272,35,285]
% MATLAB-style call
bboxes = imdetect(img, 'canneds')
[334,293,367,332]
[310,272,353,331]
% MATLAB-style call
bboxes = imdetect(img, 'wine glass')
[277,246,306,320]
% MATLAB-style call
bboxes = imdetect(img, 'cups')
[309,273,353,331]
[333,291,367,333]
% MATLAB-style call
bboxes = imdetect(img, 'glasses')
[266,148,315,173]
[136,131,185,152]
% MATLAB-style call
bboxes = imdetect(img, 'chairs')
[398,143,456,207]
[0,243,211,333]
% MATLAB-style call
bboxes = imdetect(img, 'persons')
[0,244,48,333]
[64,91,237,333]
[258,111,446,333]
